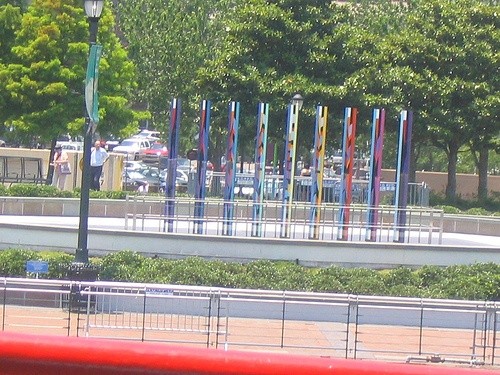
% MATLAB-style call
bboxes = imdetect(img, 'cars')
[53,130,370,198]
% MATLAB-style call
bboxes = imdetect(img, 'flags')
[84,45,105,126]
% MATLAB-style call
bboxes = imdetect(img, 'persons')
[49,143,71,190]
[90,139,110,192]
[300,162,311,177]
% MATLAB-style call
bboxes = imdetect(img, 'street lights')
[70,0,104,279]
